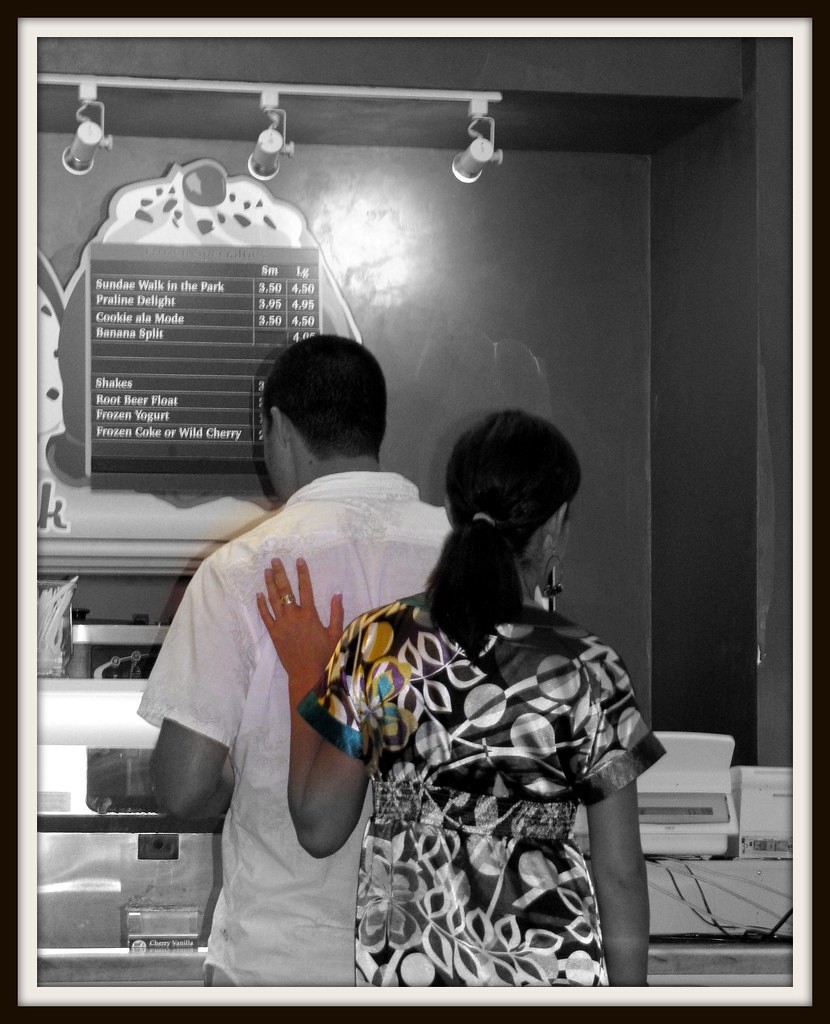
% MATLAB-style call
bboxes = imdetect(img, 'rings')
[280,594,295,607]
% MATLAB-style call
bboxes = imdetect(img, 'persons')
[138,334,453,987]
[255,408,667,986]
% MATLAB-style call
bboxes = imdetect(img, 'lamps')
[62,83,113,175]
[451,101,504,184]
[248,92,294,180]
